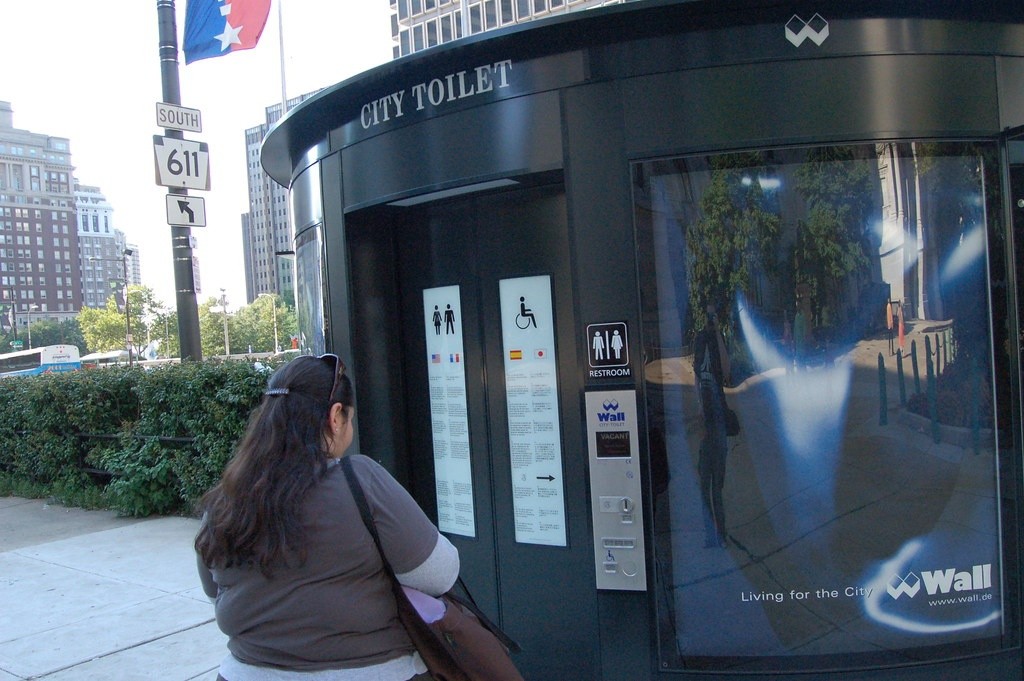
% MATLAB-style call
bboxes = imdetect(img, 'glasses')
[293,353,346,404]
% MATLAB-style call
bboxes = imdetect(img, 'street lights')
[257,293,278,355]
[130,289,151,361]
[27,305,39,350]
[121,249,134,366]
[219,288,230,356]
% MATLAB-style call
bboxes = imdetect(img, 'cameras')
[705,313,716,325]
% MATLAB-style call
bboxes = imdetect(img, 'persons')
[896,300,905,357]
[277,344,282,352]
[783,308,794,373]
[291,337,298,349]
[794,297,807,372]
[193,354,460,681]
[819,288,835,370]
[886,298,895,356]
[692,303,733,549]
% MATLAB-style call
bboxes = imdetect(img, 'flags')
[181,0,271,65]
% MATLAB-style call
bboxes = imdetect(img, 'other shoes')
[703,538,716,548]
[717,535,728,548]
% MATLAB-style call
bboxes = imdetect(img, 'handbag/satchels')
[392,573,523,681]
[723,407,741,436]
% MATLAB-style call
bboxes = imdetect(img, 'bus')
[0,344,81,377]
